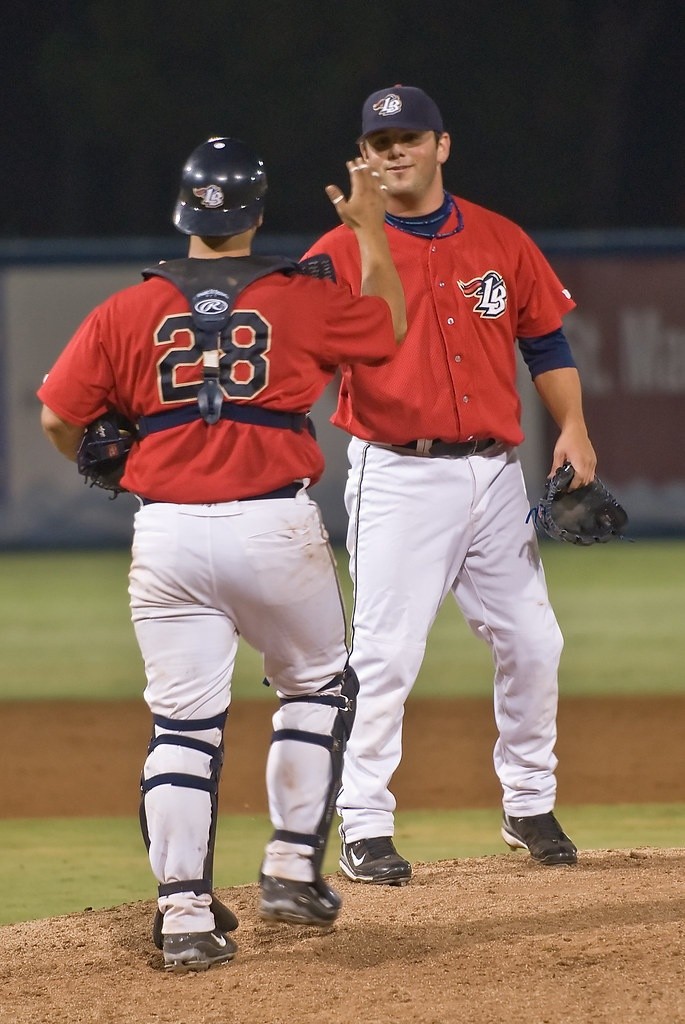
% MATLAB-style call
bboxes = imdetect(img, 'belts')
[392,439,496,457]
[141,481,303,506]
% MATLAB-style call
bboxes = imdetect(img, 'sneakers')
[339,836,412,883]
[259,875,341,928]
[501,809,578,865]
[163,929,239,970]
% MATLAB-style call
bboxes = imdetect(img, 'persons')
[37,138,407,973]
[300,85,595,885]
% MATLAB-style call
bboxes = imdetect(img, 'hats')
[356,83,443,145]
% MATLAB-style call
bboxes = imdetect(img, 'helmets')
[174,137,268,237]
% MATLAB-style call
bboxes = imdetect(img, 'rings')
[380,185,387,189]
[371,171,380,176]
[349,164,368,172]
[332,194,344,204]
